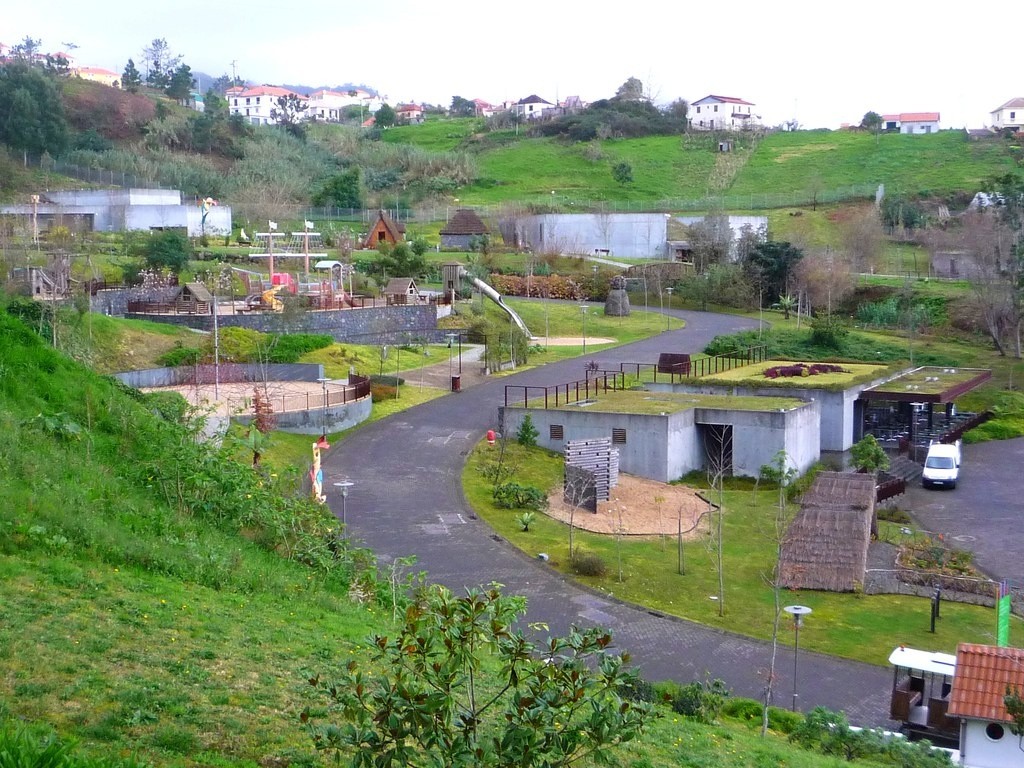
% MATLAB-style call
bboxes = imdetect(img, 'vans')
[921,440,960,490]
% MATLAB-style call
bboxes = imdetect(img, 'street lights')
[783,605,813,712]
[665,286,675,331]
[333,478,355,542]
[445,332,458,391]
[579,305,588,354]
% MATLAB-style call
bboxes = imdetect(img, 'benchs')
[926,682,952,728]
[894,675,925,718]
